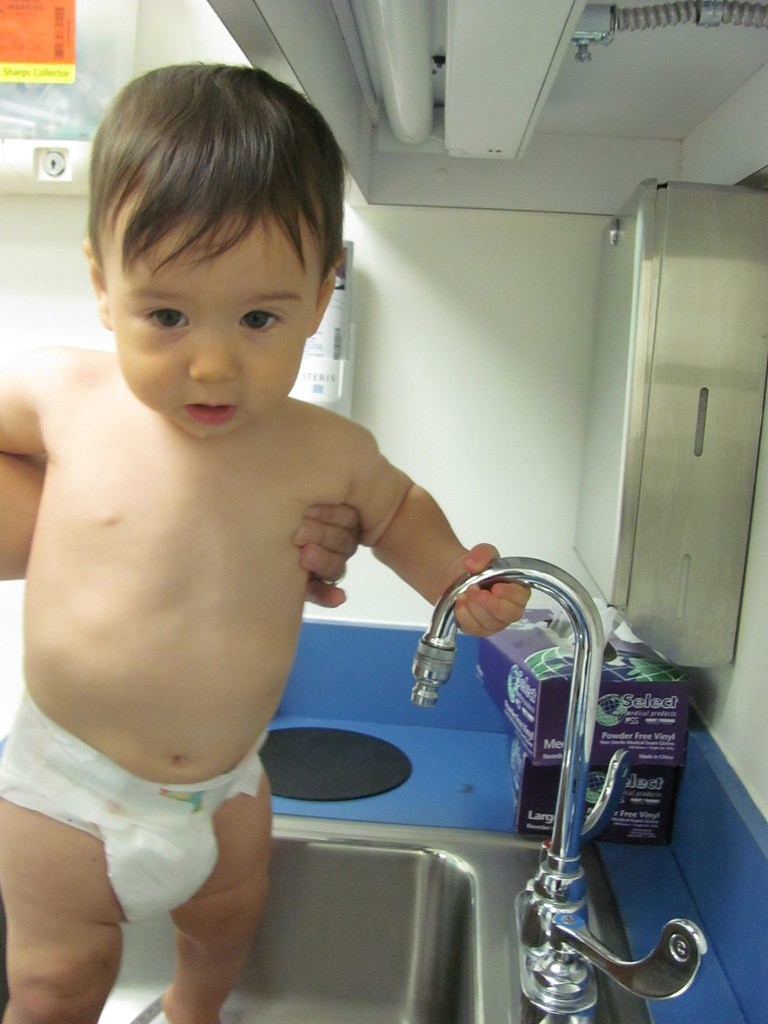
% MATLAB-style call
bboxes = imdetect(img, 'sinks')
[0,818,480,1024]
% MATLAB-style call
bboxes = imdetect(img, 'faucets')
[411,556,634,910]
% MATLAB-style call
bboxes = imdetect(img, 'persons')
[0,63,533,1021]
[0,453,361,610]
